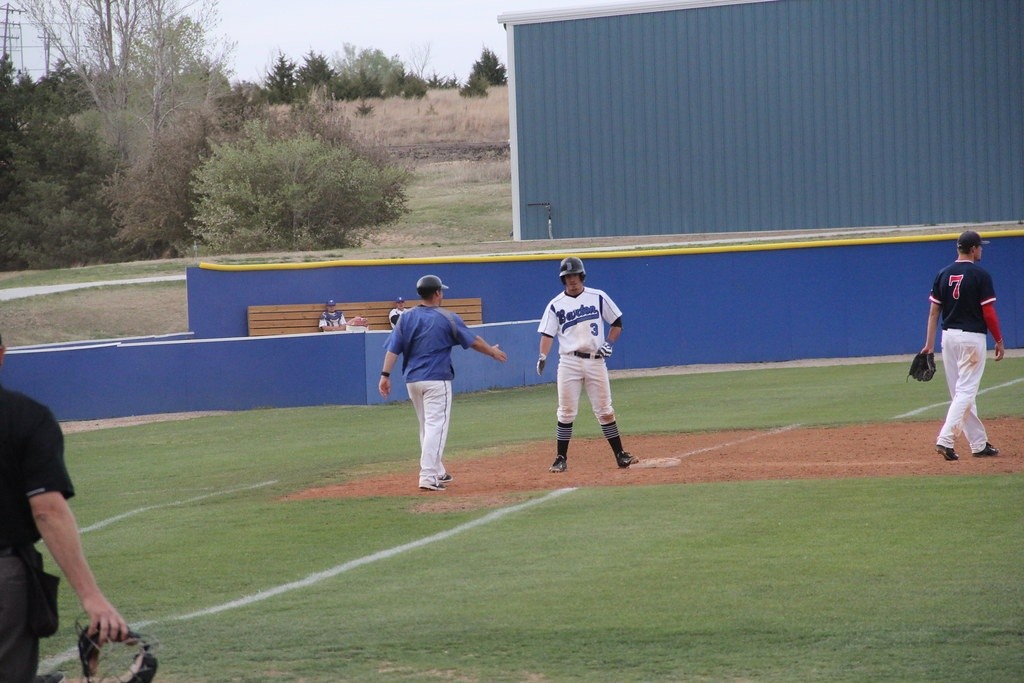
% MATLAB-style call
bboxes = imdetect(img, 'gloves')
[599,343,612,357]
[537,354,545,375]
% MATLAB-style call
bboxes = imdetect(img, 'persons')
[0,334,129,683]
[319,299,346,331]
[389,297,407,329]
[379,276,507,491]
[921,230,1004,459]
[537,257,640,471]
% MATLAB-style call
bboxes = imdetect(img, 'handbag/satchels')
[16,545,60,636]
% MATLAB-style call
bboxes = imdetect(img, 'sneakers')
[419,482,445,490]
[972,443,1000,456]
[936,444,960,461]
[550,457,566,471]
[616,453,639,468]
[438,475,453,481]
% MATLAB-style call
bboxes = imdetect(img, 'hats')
[395,297,405,301]
[326,299,336,306]
[958,231,990,245]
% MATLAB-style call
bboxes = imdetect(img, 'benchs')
[248,297,481,337]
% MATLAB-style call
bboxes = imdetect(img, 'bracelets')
[380,372,390,377]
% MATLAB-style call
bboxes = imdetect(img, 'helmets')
[416,276,448,291]
[559,258,586,284]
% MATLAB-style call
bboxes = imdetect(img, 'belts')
[574,351,601,359]
